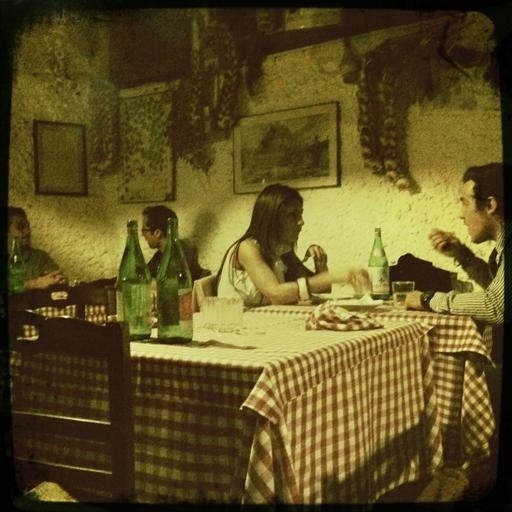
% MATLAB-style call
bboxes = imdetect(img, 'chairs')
[10,278,118,338]
[10,310,136,507]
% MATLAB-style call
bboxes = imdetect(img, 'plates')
[330,299,382,312]
[316,291,366,304]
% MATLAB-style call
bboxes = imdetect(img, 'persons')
[5,206,69,290]
[216,184,354,305]
[141,206,202,289]
[405,163,505,367]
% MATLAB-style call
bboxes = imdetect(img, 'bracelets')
[297,277,311,301]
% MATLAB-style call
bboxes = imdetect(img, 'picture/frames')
[232,101,342,194]
[33,119,90,197]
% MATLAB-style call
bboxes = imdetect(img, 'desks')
[15,311,426,505]
[242,291,493,478]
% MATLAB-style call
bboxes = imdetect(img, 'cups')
[391,280,415,311]
[200,296,245,333]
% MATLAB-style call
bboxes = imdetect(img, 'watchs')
[420,290,436,311]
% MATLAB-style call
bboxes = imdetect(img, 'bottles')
[115,216,194,344]
[6,234,25,294]
[368,227,390,300]
